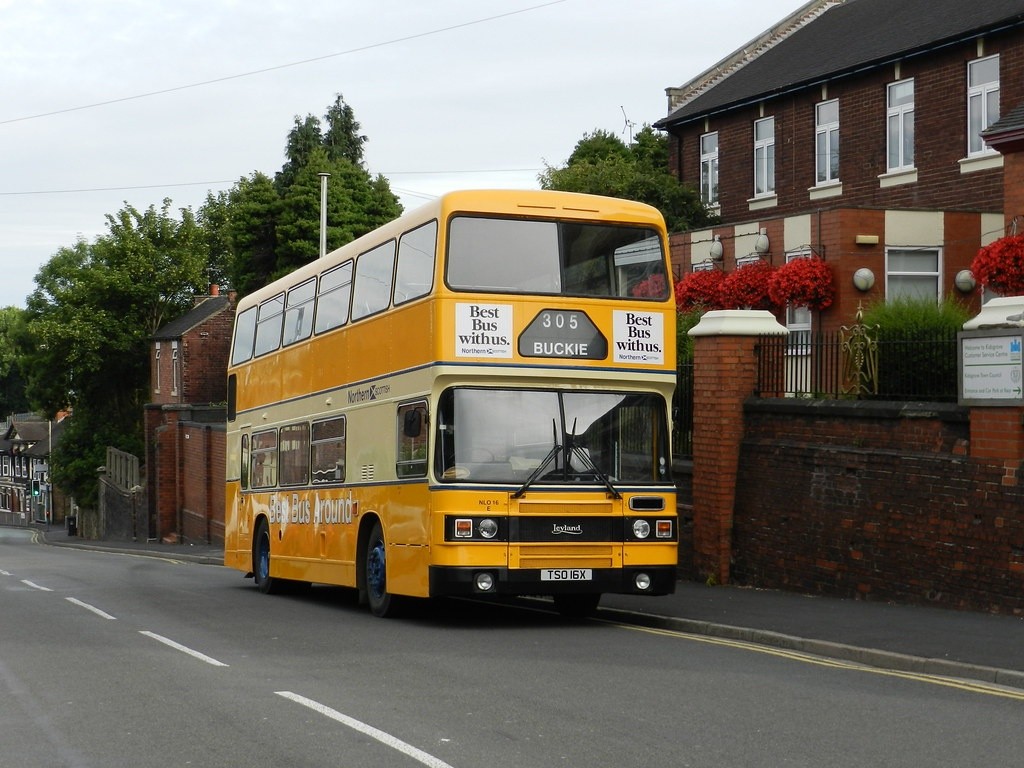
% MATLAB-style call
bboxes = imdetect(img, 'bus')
[223,188,683,622]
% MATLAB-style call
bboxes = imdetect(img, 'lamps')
[755,235,769,253]
[853,268,875,291]
[955,270,976,290]
[710,241,723,260]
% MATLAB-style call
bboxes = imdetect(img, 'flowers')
[970,230,1024,297]
[632,254,834,316]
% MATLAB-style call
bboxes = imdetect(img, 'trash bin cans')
[68,517,77,537]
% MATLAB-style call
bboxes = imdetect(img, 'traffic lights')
[32,480,41,497]
